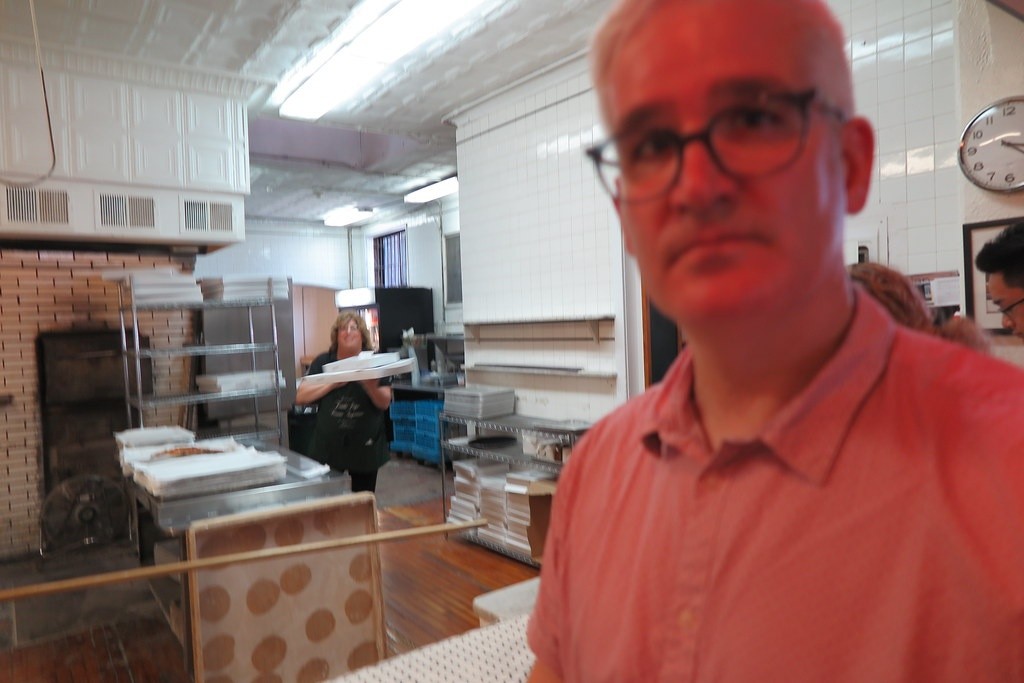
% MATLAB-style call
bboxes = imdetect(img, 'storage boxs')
[389,400,450,464]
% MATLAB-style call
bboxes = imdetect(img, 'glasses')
[583,87,851,208]
[997,298,1024,315]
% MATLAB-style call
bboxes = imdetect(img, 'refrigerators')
[335,287,434,380]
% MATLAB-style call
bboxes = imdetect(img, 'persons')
[976,223,1024,338]
[845,261,992,360]
[295,313,392,494]
[526,0,1024,682]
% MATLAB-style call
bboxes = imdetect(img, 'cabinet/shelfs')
[116,278,285,551]
[390,333,465,402]
[123,478,193,674]
[438,312,618,571]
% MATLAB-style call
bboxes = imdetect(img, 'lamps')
[404,176,458,205]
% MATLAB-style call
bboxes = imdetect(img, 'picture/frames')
[963,216,1024,336]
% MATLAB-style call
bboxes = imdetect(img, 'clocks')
[956,95,1024,193]
